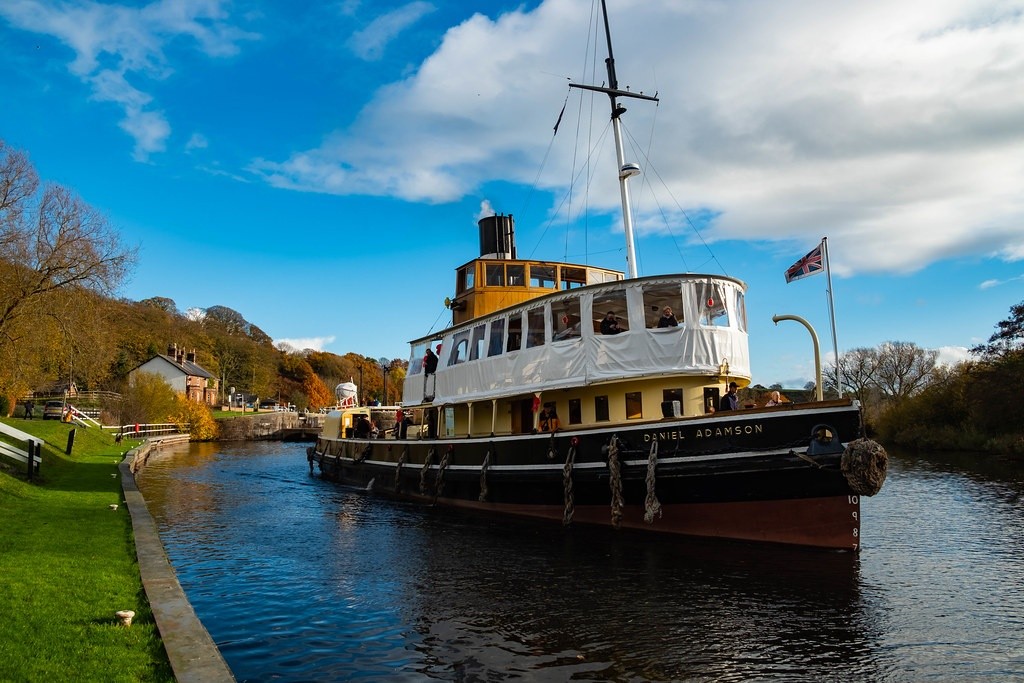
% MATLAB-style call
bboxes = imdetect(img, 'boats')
[307,0,864,554]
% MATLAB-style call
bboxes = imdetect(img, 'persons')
[357,410,408,440]
[657,305,678,328]
[600,311,621,335]
[424,349,438,377]
[537,402,561,433]
[23,400,34,420]
[765,391,782,407]
[720,382,739,411]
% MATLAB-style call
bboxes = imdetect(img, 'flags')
[784,241,825,284]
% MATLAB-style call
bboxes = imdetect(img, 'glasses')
[426,351,430,354]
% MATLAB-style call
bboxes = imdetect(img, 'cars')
[43,401,68,419]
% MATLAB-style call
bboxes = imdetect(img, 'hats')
[729,381,740,388]
[543,402,552,408]
